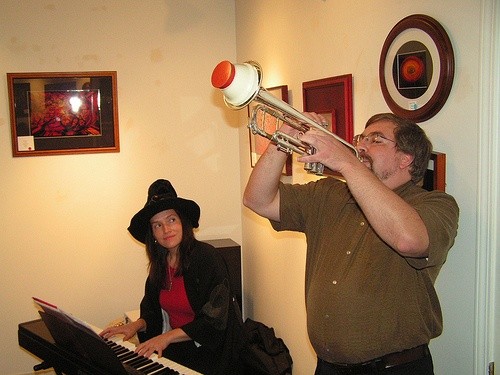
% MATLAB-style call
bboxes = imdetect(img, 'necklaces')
[167,253,178,292]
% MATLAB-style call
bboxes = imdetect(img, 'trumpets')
[213,55,361,179]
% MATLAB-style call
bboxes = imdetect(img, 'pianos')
[18,295,204,375]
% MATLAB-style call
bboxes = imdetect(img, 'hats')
[127,178,201,246]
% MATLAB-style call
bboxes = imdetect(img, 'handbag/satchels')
[242,317,292,375]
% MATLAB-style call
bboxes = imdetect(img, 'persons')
[242,113,459,375]
[99,179,230,375]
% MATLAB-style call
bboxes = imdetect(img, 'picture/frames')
[6,71,121,158]
[379,13,455,122]
[302,72,354,178]
[247,85,292,177]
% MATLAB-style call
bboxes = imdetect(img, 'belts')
[317,344,430,375]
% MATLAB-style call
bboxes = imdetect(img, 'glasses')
[351,134,396,148]
